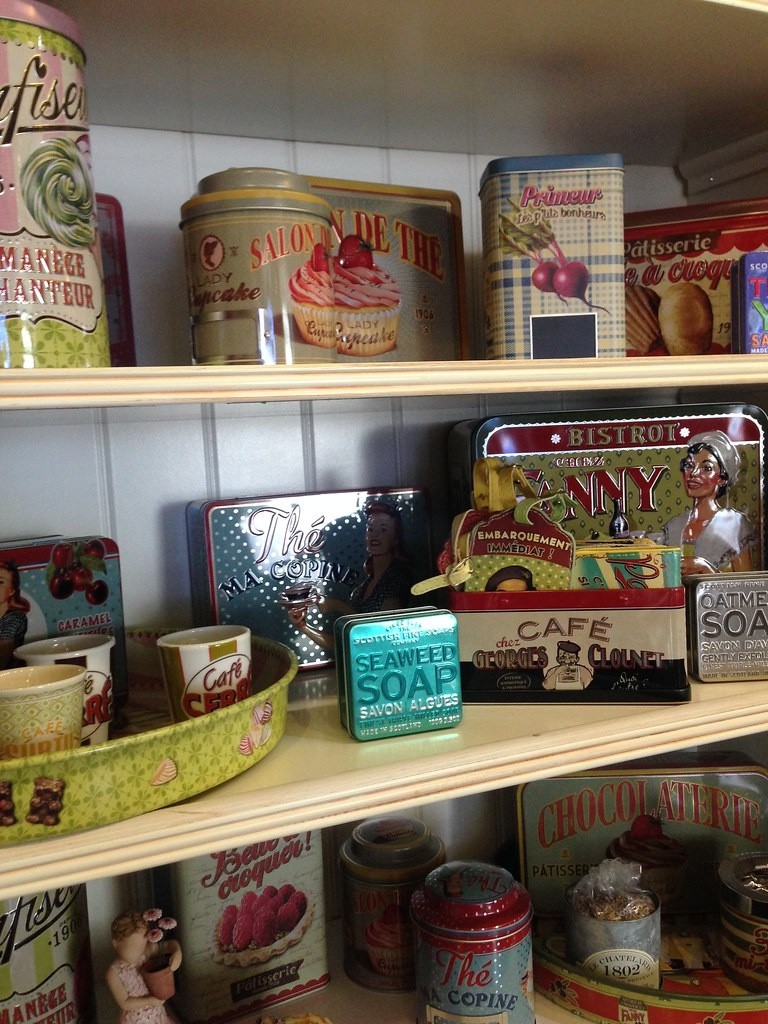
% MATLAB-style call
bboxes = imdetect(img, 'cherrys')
[48,538,108,605]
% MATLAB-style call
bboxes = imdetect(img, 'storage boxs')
[305,176,469,362]
[154,826,330,1024]
[508,749,768,1024]
[188,485,428,670]
[0,882,97,1024]
[479,152,628,358]
[335,606,462,740]
[685,570,768,682]
[97,193,137,366]
[447,583,692,707]
[0,533,130,705]
[625,197,768,354]
[448,399,768,574]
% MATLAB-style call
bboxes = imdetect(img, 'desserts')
[211,884,314,966]
[289,234,401,356]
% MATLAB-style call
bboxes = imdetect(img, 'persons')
[104,908,182,1024]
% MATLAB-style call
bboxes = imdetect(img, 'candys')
[19,138,99,249]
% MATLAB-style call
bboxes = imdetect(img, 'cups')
[563,881,661,991]
[0,665,87,762]
[12,634,116,748]
[155,625,252,724]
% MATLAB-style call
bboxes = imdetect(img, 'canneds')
[716,850,768,994]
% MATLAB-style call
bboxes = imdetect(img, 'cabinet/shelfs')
[0,0,768,1024]
